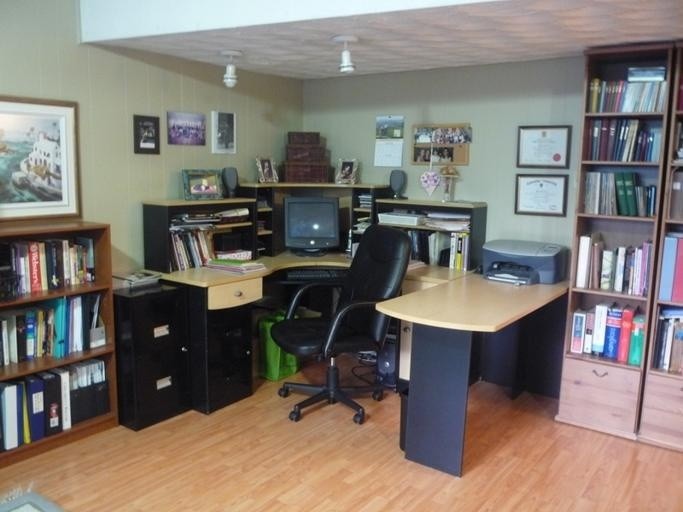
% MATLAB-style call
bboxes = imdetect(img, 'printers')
[472,240,566,287]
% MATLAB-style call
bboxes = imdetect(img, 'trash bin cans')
[377,334,399,388]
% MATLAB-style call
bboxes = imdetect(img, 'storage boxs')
[282,162,331,185]
[287,131,319,145]
[68,380,111,425]
[284,144,326,162]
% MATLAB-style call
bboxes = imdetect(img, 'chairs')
[268,223,411,425]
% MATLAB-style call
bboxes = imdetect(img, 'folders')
[0,368,72,451]
[671,238,683,303]
[658,237,677,301]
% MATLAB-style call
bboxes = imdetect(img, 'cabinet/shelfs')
[238,179,391,259]
[374,194,486,273]
[0,222,118,466]
[141,196,260,270]
[110,283,193,432]
[555,37,673,454]
[637,38,682,450]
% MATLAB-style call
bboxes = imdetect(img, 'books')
[351,193,471,279]
[0,235,114,456]
[164,203,272,279]
[564,51,681,379]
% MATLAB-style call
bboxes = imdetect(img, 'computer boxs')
[375,327,398,392]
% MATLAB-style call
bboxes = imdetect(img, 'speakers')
[390,170,408,199]
[223,167,237,198]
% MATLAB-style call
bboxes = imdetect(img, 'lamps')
[332,34,359,76]
[223,49,242,88]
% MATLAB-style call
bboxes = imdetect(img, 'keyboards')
[286,270,336,280]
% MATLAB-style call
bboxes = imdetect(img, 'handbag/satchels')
[258,310,301,381]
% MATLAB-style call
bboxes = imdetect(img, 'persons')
[411,125,472,162]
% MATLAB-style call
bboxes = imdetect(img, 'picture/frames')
[515,124,572,170]
[132,113,162,155]
[514,174,569,217]
[334,157,358,183]
[256,156,277,183]
[1,96,83,224]
[183,167,225,200]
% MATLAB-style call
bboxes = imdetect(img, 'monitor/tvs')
[282,197,340,257]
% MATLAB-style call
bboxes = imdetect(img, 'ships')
[13,130,62,201]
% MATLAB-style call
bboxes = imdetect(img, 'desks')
[140,247,480,416]
[373,270,569,480]
